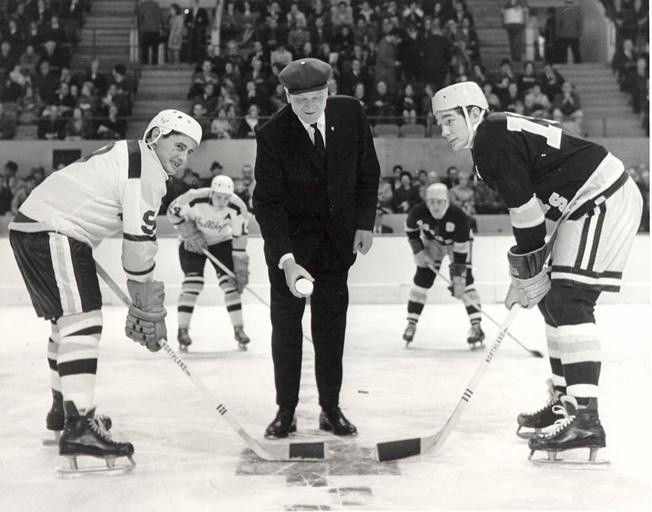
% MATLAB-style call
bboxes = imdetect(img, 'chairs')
[13,125,39,140]
[374,124,442,138]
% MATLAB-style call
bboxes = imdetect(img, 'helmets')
[431,81,489,114]
[142,109,203,148]
[211,174,234,196]
[426,183,449,200]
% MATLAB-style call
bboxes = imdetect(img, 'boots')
[528,395,605,450]
[517,379,566,428]
[46,389,133,457]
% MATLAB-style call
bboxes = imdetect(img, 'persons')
[374,164,510,234]
[1,2,649,137]
[628,161,649,232]
[159,164,256,216]
[253,58,381,440]
[8,111,201,473]
[167,175,250,353]
[401,184,485,351]
[1,160,46,216]
[430,81,644,464]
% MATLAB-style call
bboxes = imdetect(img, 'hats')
[279,57,332,94]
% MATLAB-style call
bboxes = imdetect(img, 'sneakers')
[178,328,191,345]
[403,324,416,341]
[467,324,484,343]
[234,325,249,343]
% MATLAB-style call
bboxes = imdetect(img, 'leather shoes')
[319,407,356,436]
[264,411,297,439]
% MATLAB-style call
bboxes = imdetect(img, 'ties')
[310,123,324,152]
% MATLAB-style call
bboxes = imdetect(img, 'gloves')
[232,255,249,293]
[177,220,208,255]
[446,264,467,298]
[505,243,551,310]
[415,249,433,269]
[125,279,167,352]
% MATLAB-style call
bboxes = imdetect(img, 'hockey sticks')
[429,263,543,357]
[93,260,330,462]
[370,304,523,462]
